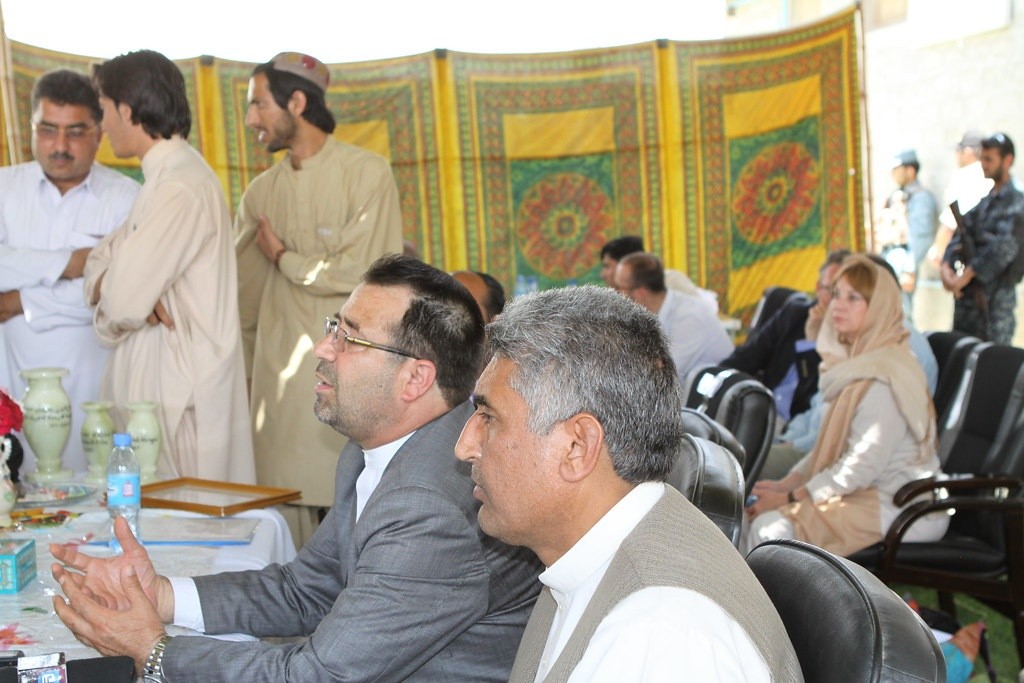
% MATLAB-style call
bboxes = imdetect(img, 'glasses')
[32,121,99,140]
[325,317,422,361]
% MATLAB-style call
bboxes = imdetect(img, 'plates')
[15,483,97,508]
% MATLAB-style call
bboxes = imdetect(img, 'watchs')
[143,635,172,683]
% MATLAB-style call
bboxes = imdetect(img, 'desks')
[0,471,297,660]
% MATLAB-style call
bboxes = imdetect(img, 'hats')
[271,52,330,92]
[956,131,986,148]
[890,149,917,169]
[993,133,1013,152]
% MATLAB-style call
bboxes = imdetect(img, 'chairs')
[668,287,1024,682]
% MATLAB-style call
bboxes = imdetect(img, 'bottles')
[106,433,142,556]
[18,368,161,477]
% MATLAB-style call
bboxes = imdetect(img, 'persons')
[939,132,1024,347]
[598,236,734,396]
[225,50,404,646]
[0,68,142,485]
[83,50,257,487]
[46,252,545,683]
[875,147,939,403]
[452,284,807,683]
[452,270,506,408]
[716,248,954,558]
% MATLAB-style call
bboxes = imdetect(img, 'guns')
[950,198,994,341]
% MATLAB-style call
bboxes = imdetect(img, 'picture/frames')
[139,476,304,520]
